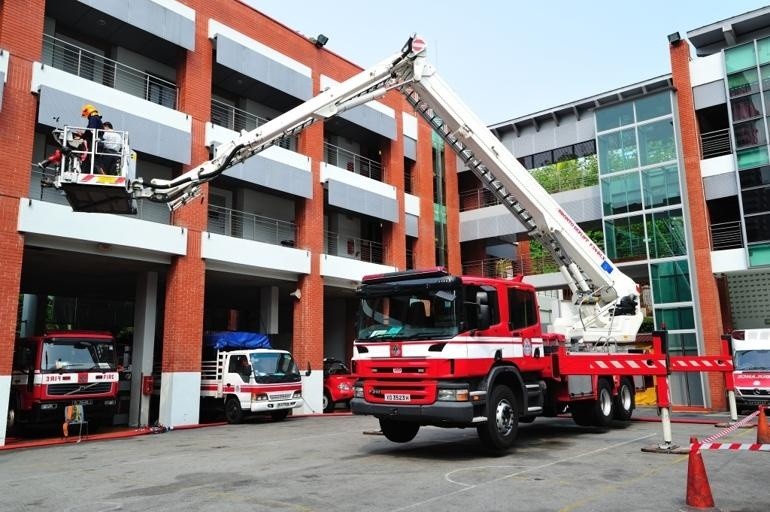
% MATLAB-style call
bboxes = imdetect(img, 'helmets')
[81,105,98,119]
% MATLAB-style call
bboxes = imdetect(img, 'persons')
[73,103,123,175]
[238,356,250,371]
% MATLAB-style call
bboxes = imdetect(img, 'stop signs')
[411,37,428,57]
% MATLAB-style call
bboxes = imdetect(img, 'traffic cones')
[755,403,770,443]
[683,434,718,508]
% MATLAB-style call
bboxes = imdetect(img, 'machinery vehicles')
[49,31,755,456]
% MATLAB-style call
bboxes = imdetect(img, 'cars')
[323,354,357,412]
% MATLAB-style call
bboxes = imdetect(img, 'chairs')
[64,405,89,442]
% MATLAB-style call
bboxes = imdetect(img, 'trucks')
[118,326,315,425]
[7,328,122,433]
[719,326,770,415]
